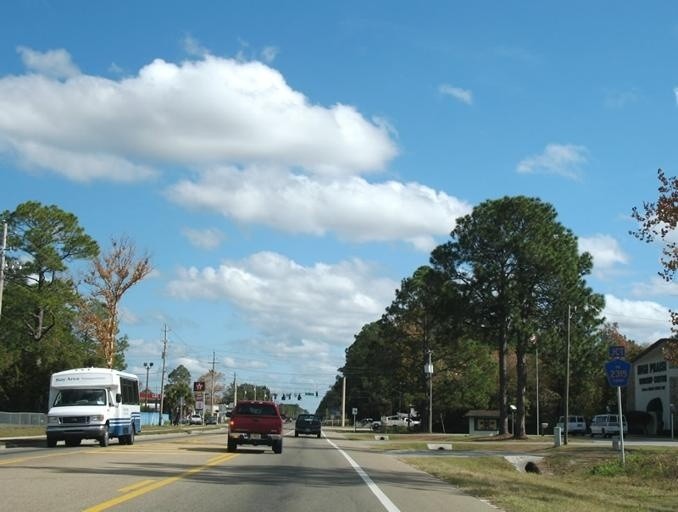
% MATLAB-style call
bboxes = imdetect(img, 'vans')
[45,367,142,447]
[555,414,629,438]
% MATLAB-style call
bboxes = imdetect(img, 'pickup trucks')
[225,400,286,455]
[294,414,322,438]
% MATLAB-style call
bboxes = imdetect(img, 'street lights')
[143,362,153,410]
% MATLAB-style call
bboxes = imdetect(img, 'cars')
[357,416,421,430]
[189,415,202,425]
[205,416,217,425]
